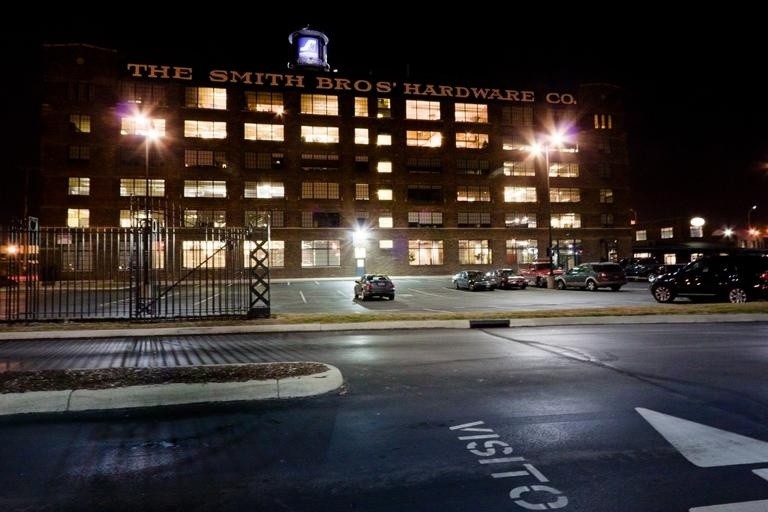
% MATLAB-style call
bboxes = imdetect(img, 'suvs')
[649,247,768,307]
[618,258,665,283]
[554,262,627,291]
[516,261,562,287]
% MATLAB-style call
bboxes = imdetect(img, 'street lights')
[131,114,157,284]
[525,126,570,291]
[747,206,756,228]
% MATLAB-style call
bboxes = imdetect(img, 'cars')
[486,268,528,290]
[353,274,394,300]
[451,270,494,291]
